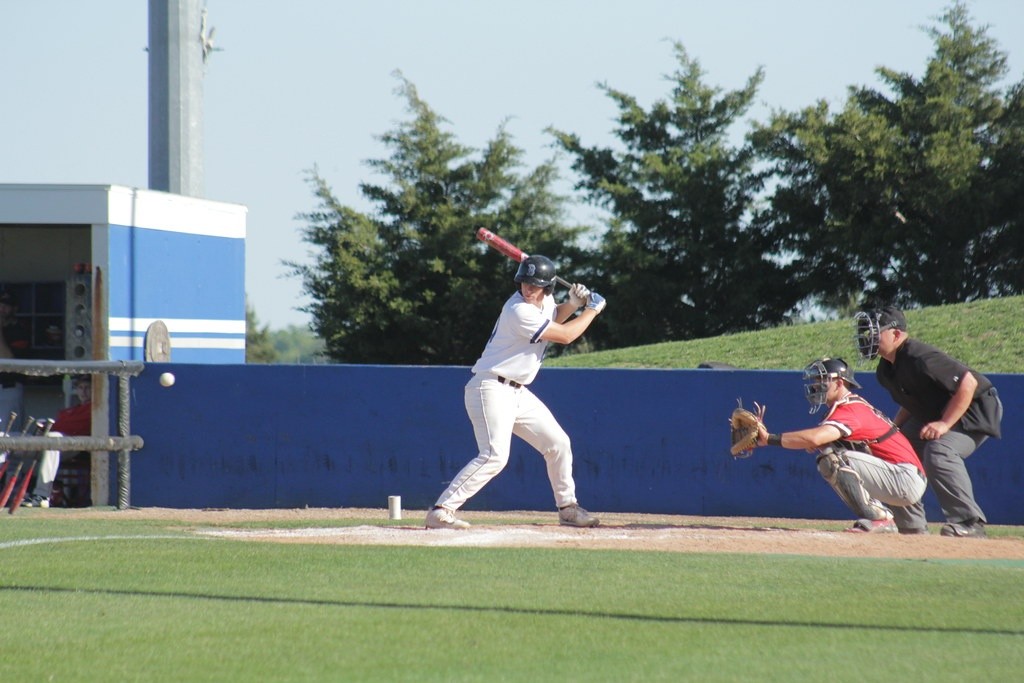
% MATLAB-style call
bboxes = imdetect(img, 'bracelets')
[766,433,782,448]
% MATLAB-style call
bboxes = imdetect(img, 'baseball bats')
[475,225,608,311]
[0,411,56,515]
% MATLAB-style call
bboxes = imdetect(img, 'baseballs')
[159,372,176,387]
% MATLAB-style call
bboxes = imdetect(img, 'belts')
[498,376,521,389]
[916,466,926,482]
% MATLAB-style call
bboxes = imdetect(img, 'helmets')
[808,356,863,390]
[513,255,557,296]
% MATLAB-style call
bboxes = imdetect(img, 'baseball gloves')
[727,395,766,461]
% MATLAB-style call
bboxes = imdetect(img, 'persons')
[0,291,30,431]
[854,307,1004,539]
[426,254,606,529]
[0,374,91,508]
[724,356,927,536]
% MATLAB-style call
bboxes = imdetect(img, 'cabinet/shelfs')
[0,279,65,349]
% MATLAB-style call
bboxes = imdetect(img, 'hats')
[860,304,906,331]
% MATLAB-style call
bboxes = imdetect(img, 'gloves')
[584,292,607,313]
[569,282,589,307]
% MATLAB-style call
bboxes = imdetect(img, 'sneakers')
[558,504,599,527]
[425,506,471,531]
[896,523,932,536]
[842,518,899,534]
[940,518,987,538]
